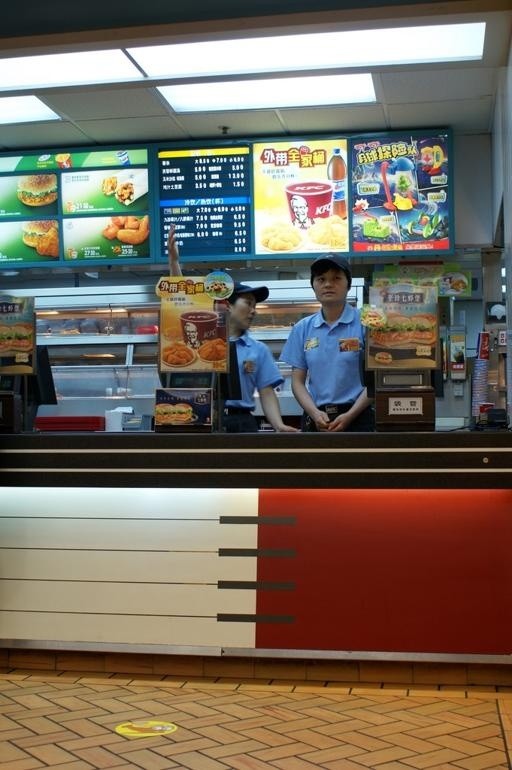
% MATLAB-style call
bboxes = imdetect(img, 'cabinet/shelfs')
[3,275,369,434]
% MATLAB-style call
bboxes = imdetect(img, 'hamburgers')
[375,351,391,364]
[365,311,437,346]
[23,220,57,247]
[0,326,33,352]
[17,174,57,206]
[155,404,192,423]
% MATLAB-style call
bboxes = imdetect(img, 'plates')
[160,344,227,368]
[260,240,305,253]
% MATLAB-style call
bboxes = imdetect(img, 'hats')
[233,282,269,303]
[312,253,350,275]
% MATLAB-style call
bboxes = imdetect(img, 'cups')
[116,151,131,166]
[53,153,73,168]
[470,330,494,427]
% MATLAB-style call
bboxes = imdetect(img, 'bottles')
[214,301,227,343]
[328,147,349,221]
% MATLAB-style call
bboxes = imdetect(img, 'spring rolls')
[102,169,148,205]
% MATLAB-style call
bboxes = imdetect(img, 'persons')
[183,323,200,348]
[279,252,374,432]
[167,222,298,432]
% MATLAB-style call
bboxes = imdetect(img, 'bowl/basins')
[155,411,199,425]
